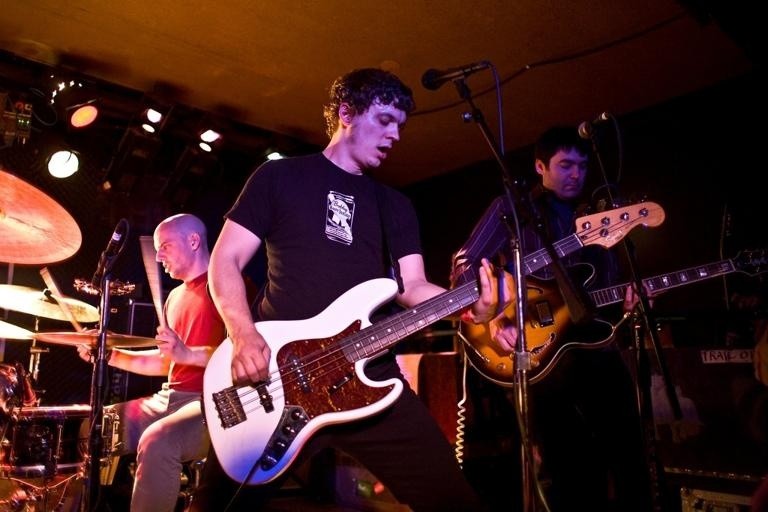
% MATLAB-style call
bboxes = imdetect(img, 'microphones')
[91,222,125,287]
[421,60,491,91]
[578,111,610,139]
[15,362,37,406]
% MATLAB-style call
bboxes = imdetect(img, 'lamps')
[49,73,99,130]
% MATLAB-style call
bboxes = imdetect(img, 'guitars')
[203,194,666,487]
[457,247,767,388]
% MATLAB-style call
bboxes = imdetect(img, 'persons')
[187,69,477,512]
[454,124,644,511]
[77,213,226,512]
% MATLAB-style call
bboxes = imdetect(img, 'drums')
[11,404,120,470]
[0,463,88,512]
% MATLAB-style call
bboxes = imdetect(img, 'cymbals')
[1,319,36,340]
[0,283,101,324]
[1,170,83,266]
[32,329,169,348]
